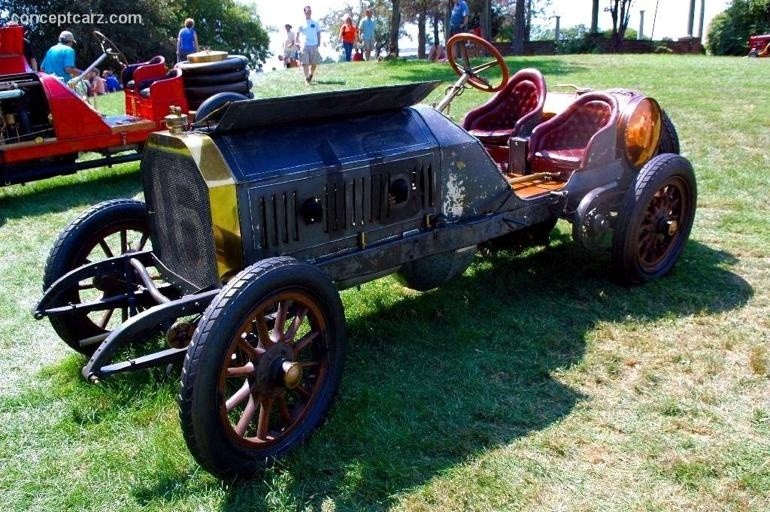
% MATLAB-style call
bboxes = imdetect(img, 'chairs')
[120,54,183,115]
[462,66,622,180]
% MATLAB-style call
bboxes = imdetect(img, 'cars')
[745,32,770,56]
[653,44,674,54]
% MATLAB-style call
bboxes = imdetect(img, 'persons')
[80,68,121,96]
[4,22,39,75]
[295,7,322,84]
[428,44,446,61]
[176,19,199,62]
[448,0,470,58]
[39,31,84,92]
[278,6,376,70]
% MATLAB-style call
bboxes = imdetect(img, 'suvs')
[0,21,261,196]
[25,25,709,486]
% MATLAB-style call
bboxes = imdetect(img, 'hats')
[60,31,77,43]
